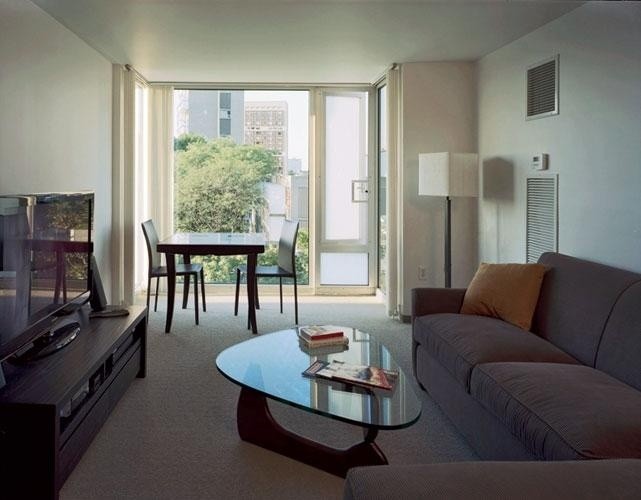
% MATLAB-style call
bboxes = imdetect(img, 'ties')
[0,190,95,363]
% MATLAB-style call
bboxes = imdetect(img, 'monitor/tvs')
[302,359,348,384]
[315,360,374,390]
[332,363,399,390]
[300,324,343,341]
[297,334,351,349]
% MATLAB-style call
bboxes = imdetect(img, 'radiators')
[88,310,130,318]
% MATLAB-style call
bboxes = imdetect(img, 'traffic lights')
[459,263,544,332]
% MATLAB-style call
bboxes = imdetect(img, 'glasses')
[419,151,479,289]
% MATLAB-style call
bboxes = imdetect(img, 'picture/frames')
[2,308,148,499]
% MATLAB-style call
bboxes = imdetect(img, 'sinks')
[412,253,641,460]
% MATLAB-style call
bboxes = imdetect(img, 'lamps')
[141,221,299,330]
